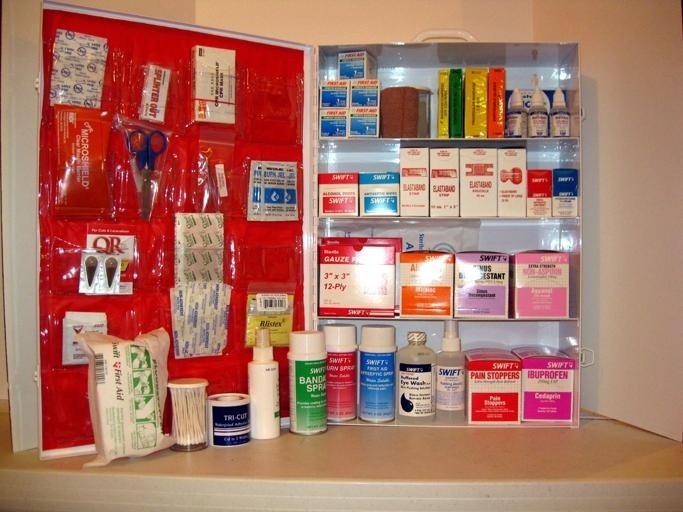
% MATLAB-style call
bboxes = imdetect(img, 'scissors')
[126,130,167,223]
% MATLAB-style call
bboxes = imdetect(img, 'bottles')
[318,324,358,423]
[359,323,397,424]
[395,330,437,418]
[505,87,529,138]
[550,84,571,136]
[286,330,328,437]
[528,73,550,138]
